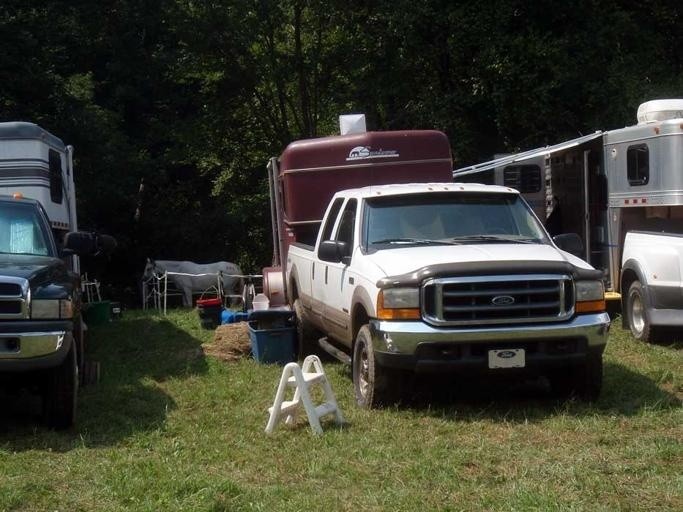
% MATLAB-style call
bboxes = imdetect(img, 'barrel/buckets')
[262,266,287,307]
[83,299,111,326]
[110,301,123,320]
[196,285,223,331]
[252,293,270,311]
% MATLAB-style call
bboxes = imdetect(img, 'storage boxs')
[298,327,332,361]
[87,299,111,322]
[244,320,297,364]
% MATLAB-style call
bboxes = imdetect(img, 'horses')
[141,257,244,309]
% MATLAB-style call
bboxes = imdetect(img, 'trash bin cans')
[110,303,123,326]
[247,310,297,364]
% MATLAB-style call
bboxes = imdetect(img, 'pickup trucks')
[0,194,92,432]
[620,227,682,345]
[284,181,611,410]
